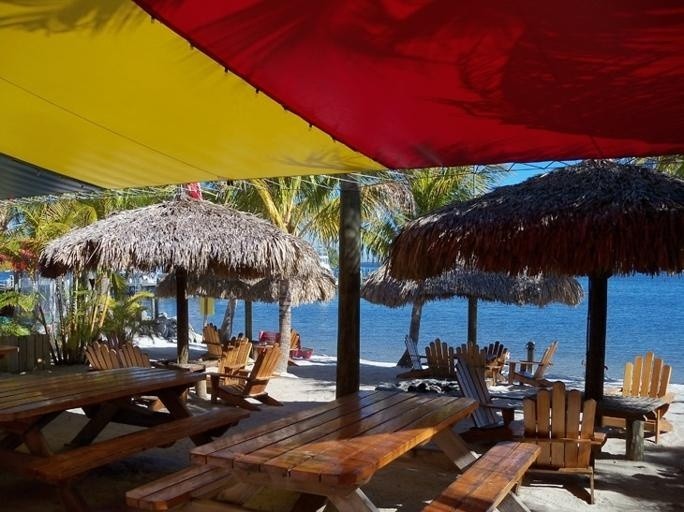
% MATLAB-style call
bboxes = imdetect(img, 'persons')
[230,336,236,340]
[237,332,243,341]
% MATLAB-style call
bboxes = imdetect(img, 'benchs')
[125,464,254,512]
[24,407,249,512]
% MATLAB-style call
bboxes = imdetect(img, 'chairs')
[82,325,315,411]
[602,353,672,443]
[517,380,610,507]
[451,357,526,448]
[405,330,508,385]
[508,342,557,389]
[421,441,540,512]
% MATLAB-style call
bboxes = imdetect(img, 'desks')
[529,387,668,461]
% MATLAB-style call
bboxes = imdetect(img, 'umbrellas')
[389,157,683,458]
[361,262,583,344]
[156,265,334,357]
[38,195,322,367]
